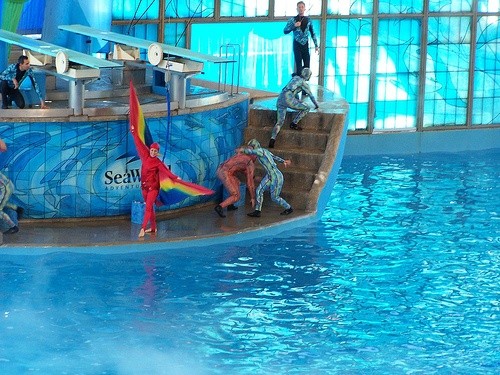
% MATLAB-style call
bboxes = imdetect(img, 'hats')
[301,67,312,80]
[149,142,160,152]
[247,138,261,150]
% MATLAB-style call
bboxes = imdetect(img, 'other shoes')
[268,139,275,148]
[1,102,8,109]
[247,210,261,217]
[214,204,227,218]
[227,203,238,211]
[289,122,302,131]
[15,206,25,219]
[7,95,13,106]
[2,226,18,234]
[280,206,293,215]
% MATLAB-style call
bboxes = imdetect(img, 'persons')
[0,56,50,110]
[214,139,293,218]
[131,127,182,237]
[269,67,320,148]
[0,140,24,234]
[284,2,320,94]
